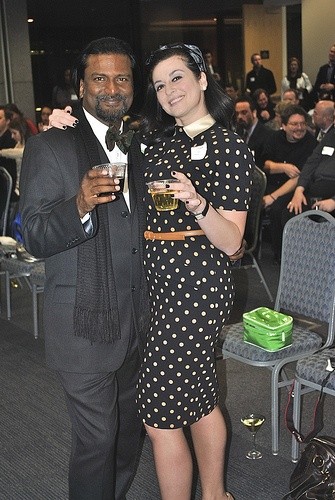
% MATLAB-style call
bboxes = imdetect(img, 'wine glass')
[240,414,265,459]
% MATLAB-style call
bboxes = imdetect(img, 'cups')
[92,162,126,197]
[145,179,179,212]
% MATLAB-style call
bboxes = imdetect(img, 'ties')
[243,130,248,143]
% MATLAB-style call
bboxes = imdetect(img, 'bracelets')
[194,199,209,220]
[270,194,276,202]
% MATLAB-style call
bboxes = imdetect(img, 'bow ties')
[105,123,134,155]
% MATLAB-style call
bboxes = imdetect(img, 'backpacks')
[281,435,335,499]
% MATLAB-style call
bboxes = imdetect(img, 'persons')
[19,37,149,500]
[0,65,78,219]
[42,41,256,500]
[202,44,335,268]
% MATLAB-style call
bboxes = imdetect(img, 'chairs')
[218,165,335,464]
[0,167,44,340]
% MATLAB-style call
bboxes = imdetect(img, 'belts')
[143,230,204,242]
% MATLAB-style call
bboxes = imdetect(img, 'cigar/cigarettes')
[316,199,319,210]
